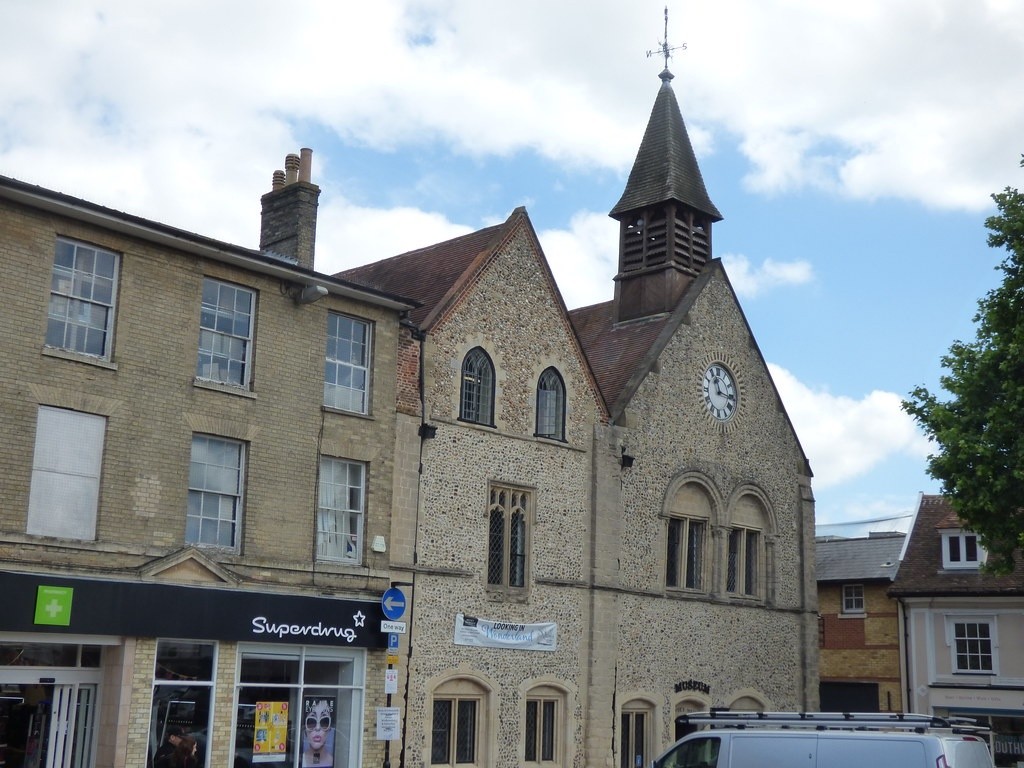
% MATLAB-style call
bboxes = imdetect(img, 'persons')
[153,724,188,768]
[302,699,334,767]
[172,737,197,768]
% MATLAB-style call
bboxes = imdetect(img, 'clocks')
[703,364,737,420]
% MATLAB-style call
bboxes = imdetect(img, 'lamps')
[290,278,328,303]
[621,445,637,470]
[418,423,437,441]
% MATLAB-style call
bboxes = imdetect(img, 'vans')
[649,709,994,768]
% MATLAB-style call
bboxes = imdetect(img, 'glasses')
[306,716,331,732]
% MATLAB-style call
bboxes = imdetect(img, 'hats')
[170,726,189,738]
